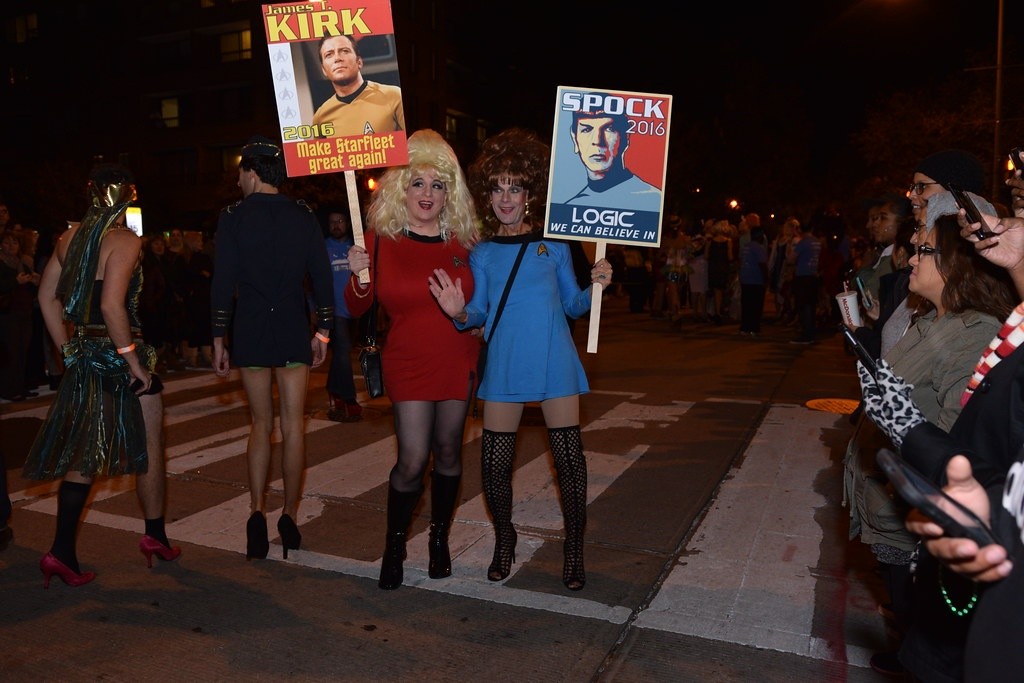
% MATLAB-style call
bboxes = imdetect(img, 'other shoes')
[869,651,913,676]
[630,306,843,345]
[0,526,13,552]
[2,391,39,402]
[166,358,216,374]
[878,600,908,643]
[50,380,60,390]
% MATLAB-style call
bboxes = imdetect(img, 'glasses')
[914,243,942,263]
[906,181,939,195]
[327,218,350,226]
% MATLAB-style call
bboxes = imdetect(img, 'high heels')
[326,384,343,407]
[41,552,95,587]
[343,396,363,415]
[245,510,270,562]
[278,514,302,560]
[137,534,181,569]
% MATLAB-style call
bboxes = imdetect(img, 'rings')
[439,289,442,294]
[601,264,603,269]
[600,274,605,279]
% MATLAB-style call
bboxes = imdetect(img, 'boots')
[427,469,463,579]
[545,425,587,590]
[480,427,518,581]
[378,463,424,589]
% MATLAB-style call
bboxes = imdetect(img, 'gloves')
[857,356,928,455]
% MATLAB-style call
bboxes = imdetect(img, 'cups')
[836,291,861,327]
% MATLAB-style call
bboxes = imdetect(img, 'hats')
[241,142,285,160]
[916,151,984,199]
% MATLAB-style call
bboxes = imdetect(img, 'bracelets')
[315,332,329,343]
[351,273,370,298]
[938,564,978,616]
[117,344,135,354]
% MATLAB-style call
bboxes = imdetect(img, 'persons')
[313,30,406,138]
[209,143,336,558]
[839,143,1024,683]
[315,202,366,419]
[562,199,967,343]
[0,449,14,552]
[38,172,181,588]
[343,129,486,590]
[0,202,262,401]
[428,130,612,591]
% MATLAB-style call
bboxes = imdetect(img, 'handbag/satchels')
[358,344,385,400]
[476,340,489,389]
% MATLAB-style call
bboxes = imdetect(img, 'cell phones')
[874,448,1000,549]
[948,182,995,240]
[841,325,876,369]
[1010,148,1024,181]
[856,276,873,308]
